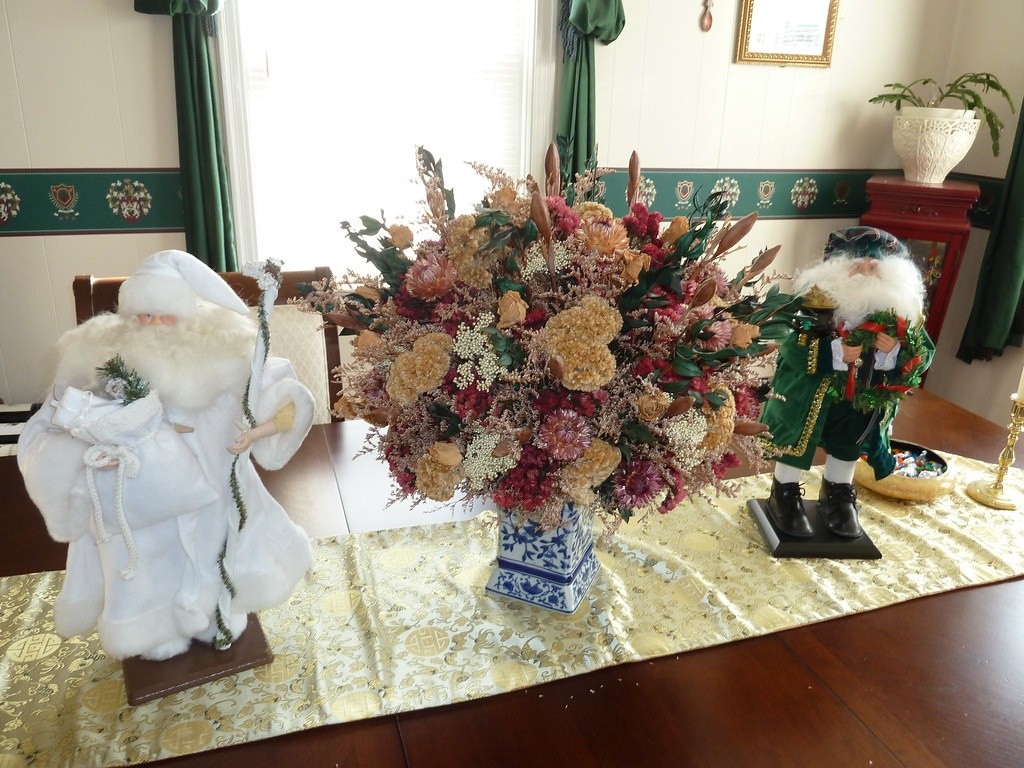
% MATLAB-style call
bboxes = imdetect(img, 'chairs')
[72,266,345,423]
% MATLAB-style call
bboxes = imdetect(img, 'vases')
[485,492,602,617]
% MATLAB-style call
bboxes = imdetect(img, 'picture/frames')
[736,0,840,69]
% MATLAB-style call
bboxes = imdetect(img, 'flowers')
[284,134,805,551]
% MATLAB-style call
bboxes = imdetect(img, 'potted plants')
[868,72,1015,184]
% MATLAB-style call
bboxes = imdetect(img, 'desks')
[0,386,1024,768]
[859,175,979,388]
